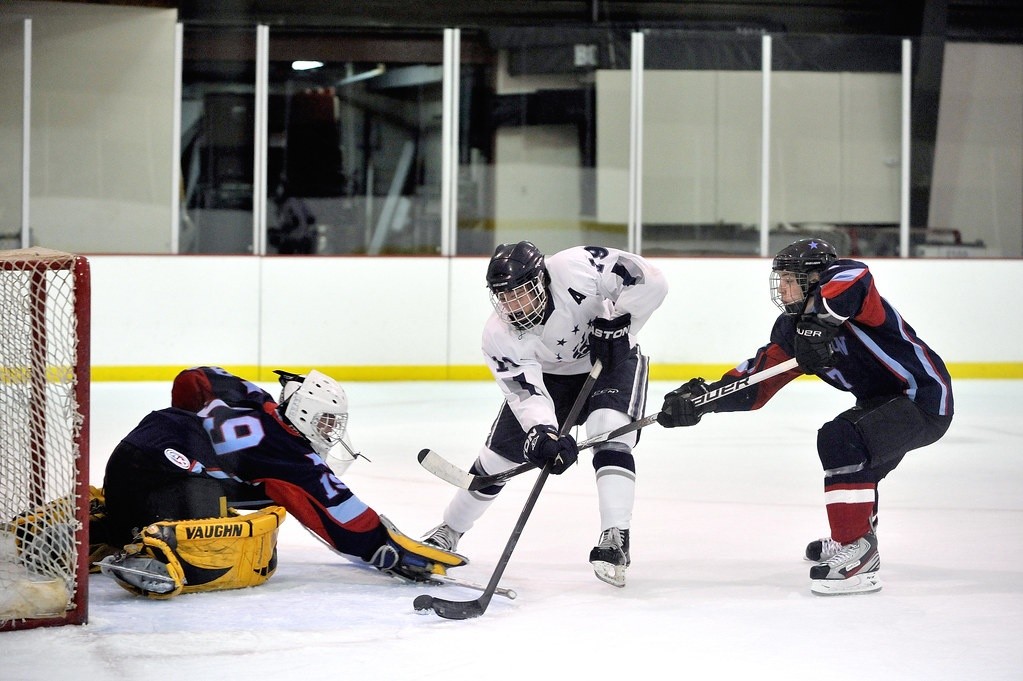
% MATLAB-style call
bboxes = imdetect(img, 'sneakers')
[810,511,882,597]
[589,528,630,588]
[418,522,465,552]
[803,516,878,563]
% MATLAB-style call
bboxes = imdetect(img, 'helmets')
[768,238,838,317]
[273,370,371,479]
[486,240,551,332]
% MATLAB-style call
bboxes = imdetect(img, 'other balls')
[413,594,434,615]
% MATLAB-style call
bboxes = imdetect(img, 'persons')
[16,364,468,600]
[424,240,668,588]
[657,238,954,596]
[267,182,318,255]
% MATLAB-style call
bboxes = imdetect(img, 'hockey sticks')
[417,342,834,493]
[432,360,603,620]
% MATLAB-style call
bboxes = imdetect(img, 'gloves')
[361,535,446,586]
[524,424,579,475]
[588,313,631,378]
[657,377,718,428]
[793,321,840,375]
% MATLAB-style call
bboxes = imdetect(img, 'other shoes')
[100,555,175,592]
[15,523,74,574]
